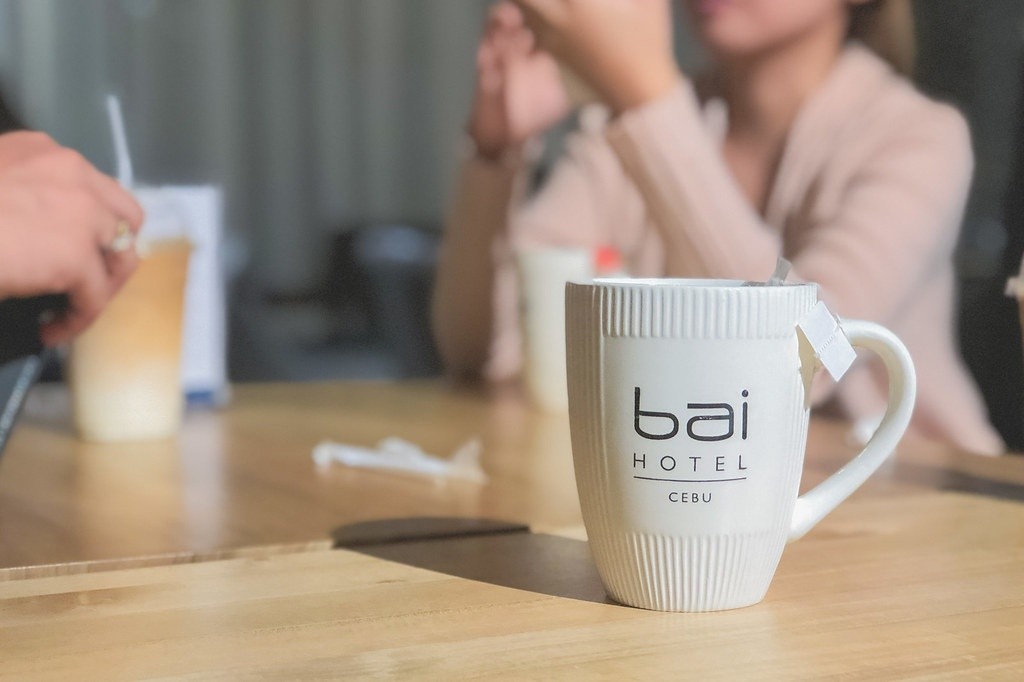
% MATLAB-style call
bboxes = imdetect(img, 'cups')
[59,237,192,438]
[563,278,917,612]
[514,248,627,417]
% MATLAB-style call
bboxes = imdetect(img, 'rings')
[100,220,135,254]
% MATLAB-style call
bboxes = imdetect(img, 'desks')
[0,384,1024,682]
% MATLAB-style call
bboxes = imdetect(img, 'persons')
[432,0,1007,459]
[0,81,144,347]
